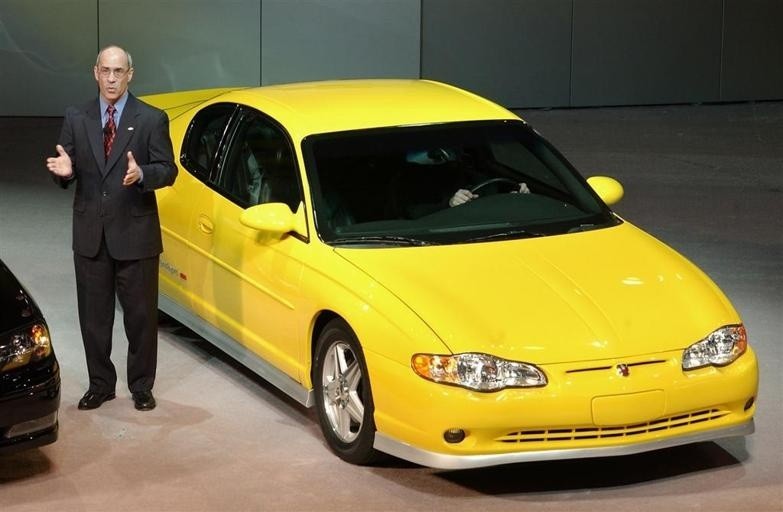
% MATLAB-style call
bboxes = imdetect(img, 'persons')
[46,46,178,411]
[407,182,531,219]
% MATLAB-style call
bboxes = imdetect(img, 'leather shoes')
[78,390,115,410]
[132,391,155,411]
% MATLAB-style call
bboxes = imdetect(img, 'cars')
[117,74,756,473]
[0,251,68,448]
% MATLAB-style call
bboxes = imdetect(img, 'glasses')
[97,64,135,78]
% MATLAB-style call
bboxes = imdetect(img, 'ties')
[104,104,116,160]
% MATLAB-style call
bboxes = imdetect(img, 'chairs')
[396,144,498,219]
